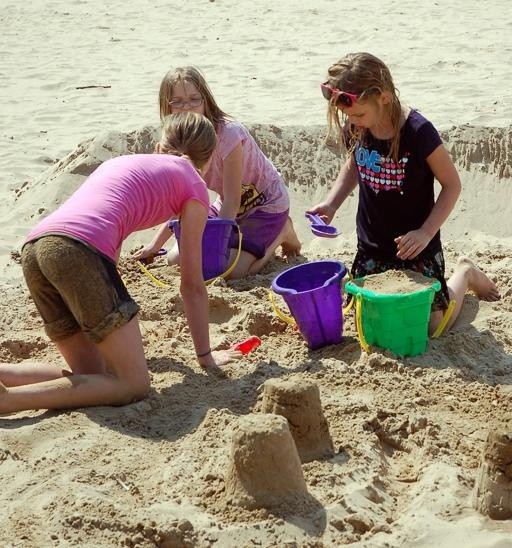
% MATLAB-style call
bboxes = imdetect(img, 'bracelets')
[196,349,213,359]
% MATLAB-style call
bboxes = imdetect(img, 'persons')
[158,66,302,280]
[305,53,500,338]
[1,113,229,413]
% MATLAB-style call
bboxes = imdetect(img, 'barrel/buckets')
[344,273,441,358]
[169,218,236,279]
[269,259,354,350]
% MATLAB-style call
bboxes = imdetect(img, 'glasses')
[321,79,357,110]
[166,97,204,110]
